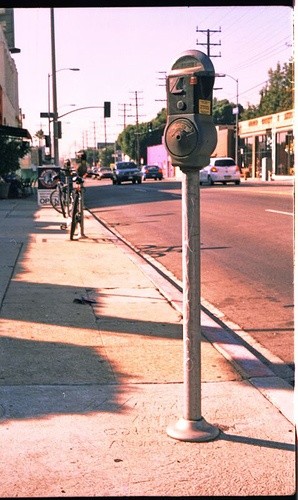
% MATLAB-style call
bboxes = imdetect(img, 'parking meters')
[163,49,218,441]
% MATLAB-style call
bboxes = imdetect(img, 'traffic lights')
[104,101,111,117]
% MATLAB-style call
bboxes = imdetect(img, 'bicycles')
[50,167,73,217]
[68,176,84,240]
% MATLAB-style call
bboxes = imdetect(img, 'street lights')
[48,67,81,165]
[220,73,240,164]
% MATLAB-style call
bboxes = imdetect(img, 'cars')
[112,162,142,185]
[200,158,240,185]
[142,165,163,180]
[97,167,113,180]
